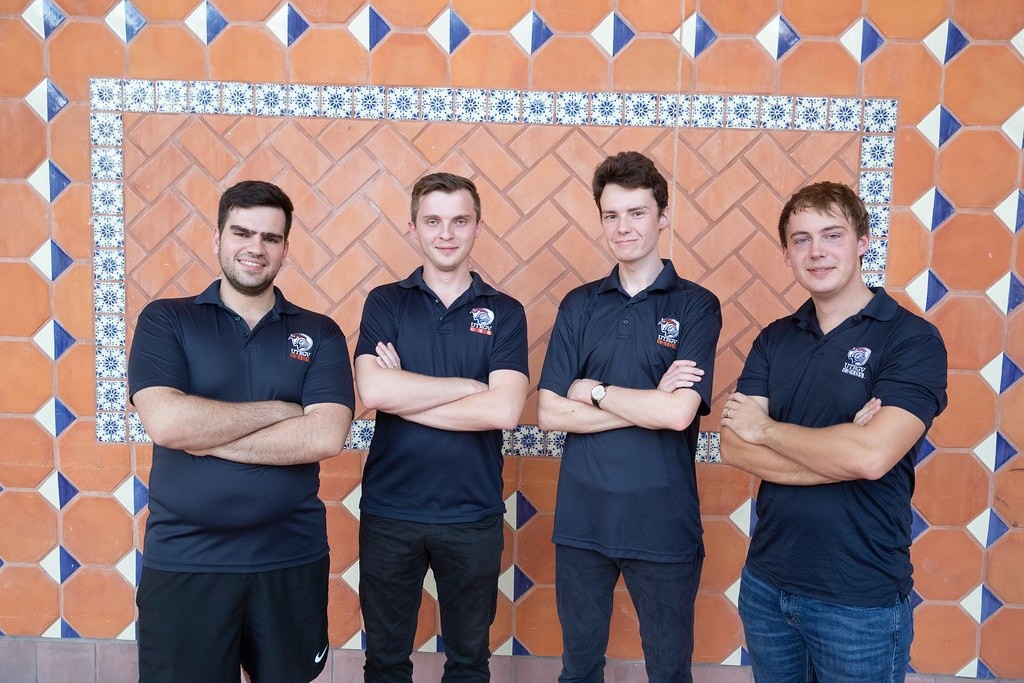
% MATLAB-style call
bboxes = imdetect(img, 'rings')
[390,365,396,369]
[726,409,732,418]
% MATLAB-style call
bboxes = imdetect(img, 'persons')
[720,183,947,683]
[353,173,528,683]
[536,152,723,682]
[128,181,355,683]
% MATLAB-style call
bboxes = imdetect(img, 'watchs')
[591,382,612,410]
